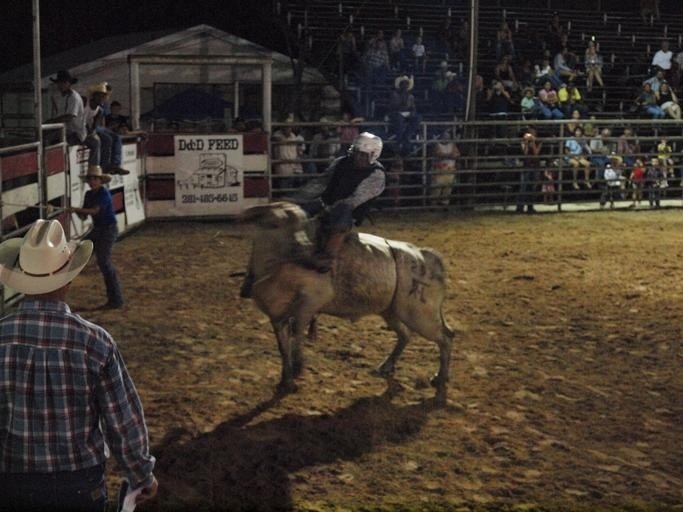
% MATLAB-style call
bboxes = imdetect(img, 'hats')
[395,75,414,91]
[78,164,110,185]
[96,81,113,94]
[523,86,535,95]
[49,70,78,85]
[0,219,93,295]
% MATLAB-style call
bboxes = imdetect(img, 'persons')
[71,165,123,309]
[44,70,144,175]
[0,218,159,512]
[233,13,683,230]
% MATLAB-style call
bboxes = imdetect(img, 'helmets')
[345,132,383,165]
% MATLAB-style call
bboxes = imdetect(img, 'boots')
[317,230,348,273]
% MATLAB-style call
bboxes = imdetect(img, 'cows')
[240,200,456,407]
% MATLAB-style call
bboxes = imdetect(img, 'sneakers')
[101,166,130,175]
[97,302,120,310]
